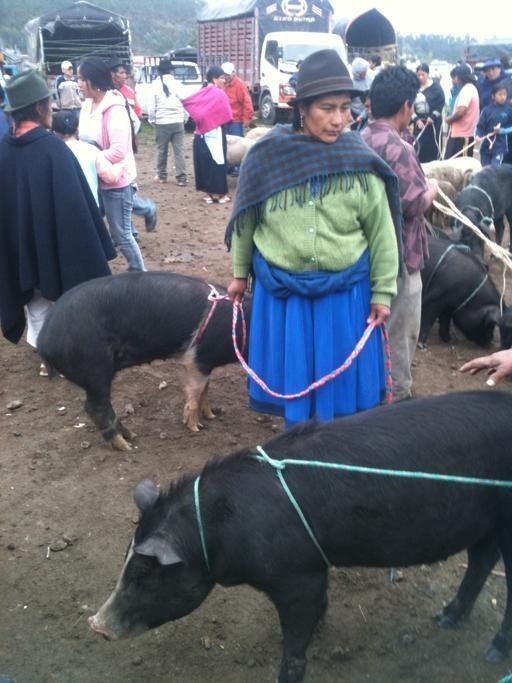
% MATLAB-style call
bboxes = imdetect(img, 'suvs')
[456,41,512,77]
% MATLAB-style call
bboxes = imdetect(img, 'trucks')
[196,0,349,127]
[330,6,399,71]
[25,2,134,88]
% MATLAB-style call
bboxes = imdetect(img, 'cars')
[130,46,203,128]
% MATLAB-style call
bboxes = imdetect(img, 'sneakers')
[144,210,157,231]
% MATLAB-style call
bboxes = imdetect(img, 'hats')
[287,49,366,107]
[3,69,58,113]
[220,61,235,75]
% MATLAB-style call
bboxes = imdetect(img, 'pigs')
[416,237,509,350]
[35,270,252,452]
[87,388,511,683]
[419,156,512,259]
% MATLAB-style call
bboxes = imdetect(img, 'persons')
[225,50,403,434]
[0,59,254,377]
[457,348,512,392]
[350,55,511,165]
[356,67,439,400]
